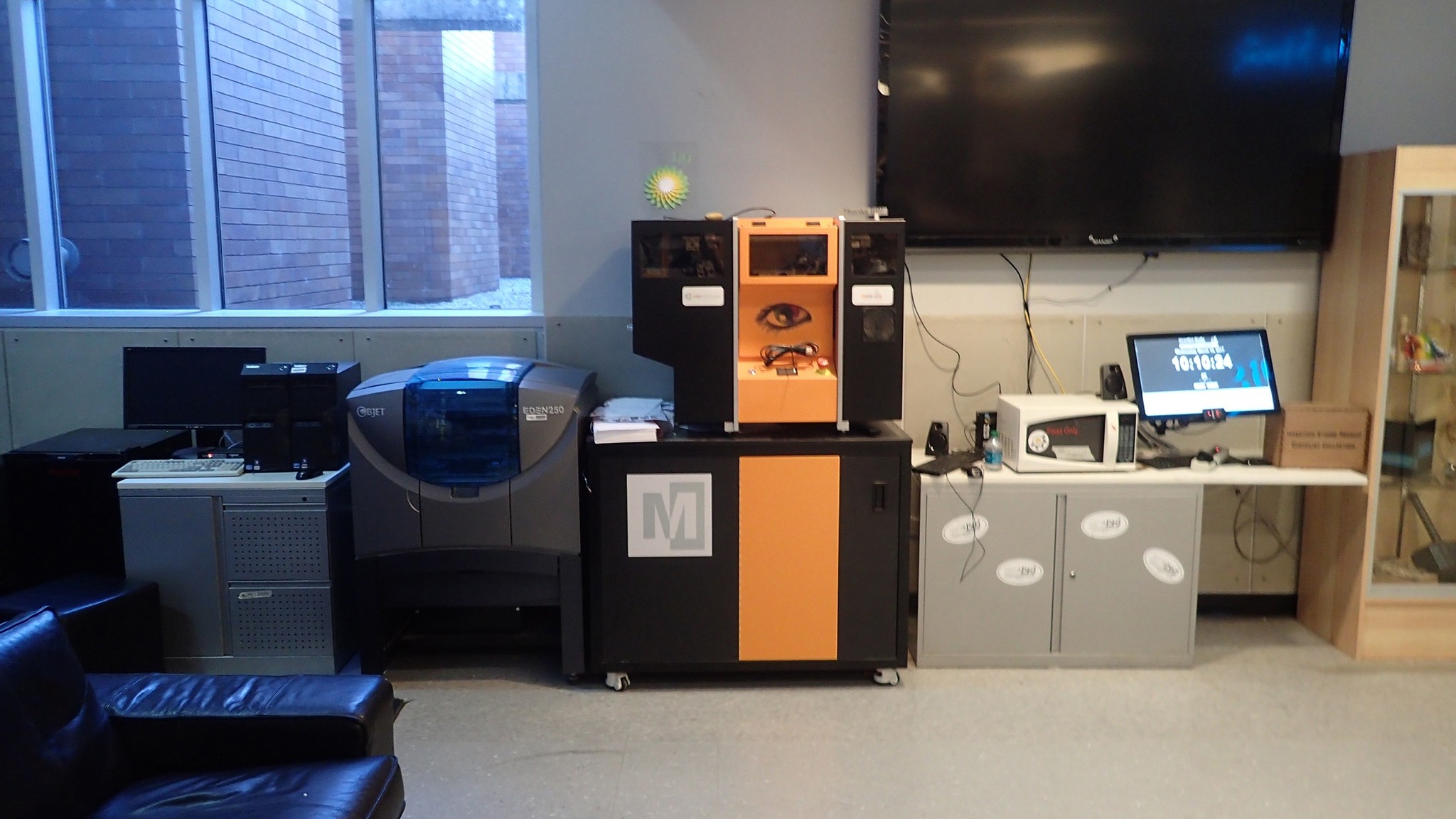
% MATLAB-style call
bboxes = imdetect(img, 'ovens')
[993,392,1139,474]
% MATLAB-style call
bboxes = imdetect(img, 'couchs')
[0,579,405,819]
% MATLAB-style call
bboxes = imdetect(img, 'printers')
[344,356,599,682]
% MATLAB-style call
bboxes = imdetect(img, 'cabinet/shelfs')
[912,448,1371,668]
[588,416,911,689]
[1297,144,1455,661]
[117,481,355,676]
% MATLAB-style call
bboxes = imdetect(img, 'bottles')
[984,430,1003,471]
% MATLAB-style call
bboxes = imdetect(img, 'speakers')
[924,421,947,455]
[1098,363,1128,400]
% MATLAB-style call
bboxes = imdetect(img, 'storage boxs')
[1264,405,1368,467]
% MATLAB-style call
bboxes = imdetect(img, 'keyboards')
[915,450,984,476]
[111,459,244,478]
[1136,454,1246,469]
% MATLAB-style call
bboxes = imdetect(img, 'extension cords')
[1191,447,1229,471]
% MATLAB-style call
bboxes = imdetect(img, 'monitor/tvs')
[1125,328,1281,428]
[877,0,1357,251]
[122,347,266,459]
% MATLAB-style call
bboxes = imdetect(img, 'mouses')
[296,468,323,481]
[967,466,982,478]
